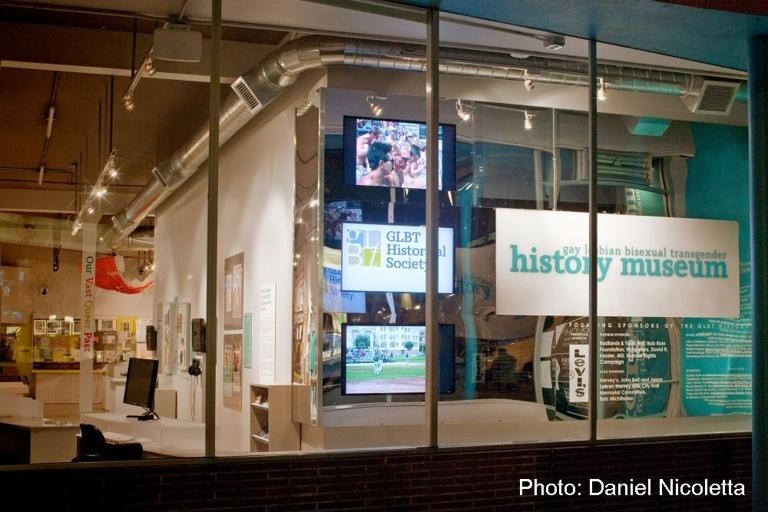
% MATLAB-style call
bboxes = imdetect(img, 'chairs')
[74,424,143,461]
[154,390,177,419]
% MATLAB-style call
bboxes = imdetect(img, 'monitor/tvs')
[191,318,206,353]
[145,325,157,351]
[341,115,457,205]
[340,220,457,296]
[339,322,456,396]
[122,356,160,421]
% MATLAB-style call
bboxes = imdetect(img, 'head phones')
[188,358,201,376]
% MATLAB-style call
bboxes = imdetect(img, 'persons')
[488,348,518,383]
[355,118,441,189]
[347,346,411,374]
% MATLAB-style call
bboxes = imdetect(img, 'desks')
[0,417,80,465]
[80,411,205,457]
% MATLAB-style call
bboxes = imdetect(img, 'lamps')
[121,18,169,112]
[366,93,537,130]
[69,75,119,236]
[523,67,607,100]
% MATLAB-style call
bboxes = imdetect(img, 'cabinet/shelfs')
[249,382,300,452]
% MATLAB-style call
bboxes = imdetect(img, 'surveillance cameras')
[137,261,153,276]
